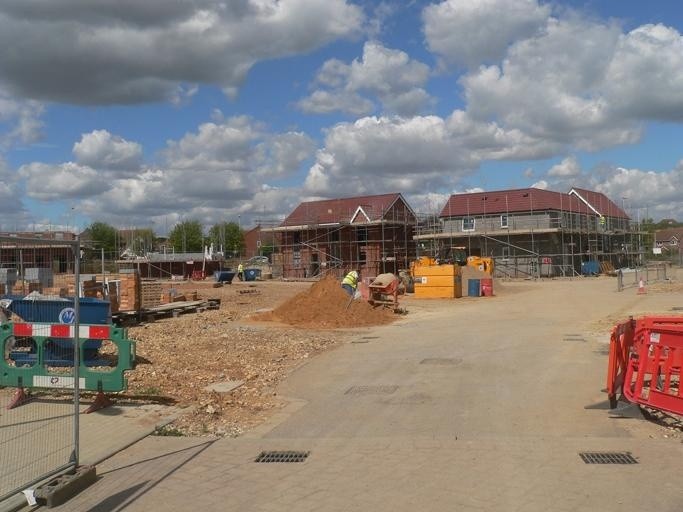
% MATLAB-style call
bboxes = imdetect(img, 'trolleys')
[367,273,400,313]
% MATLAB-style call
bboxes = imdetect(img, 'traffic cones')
[636,277,646,295]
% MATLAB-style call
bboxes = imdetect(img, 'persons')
[598,215,606,233]
[236,262,244,282]
[341,269,361,297]
